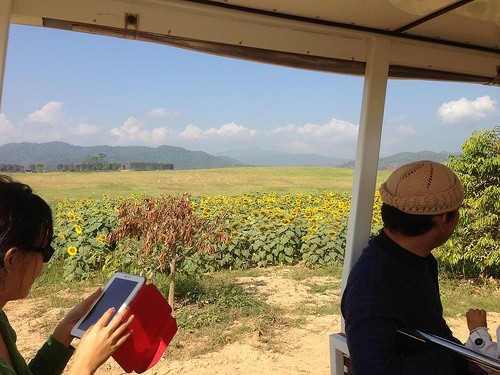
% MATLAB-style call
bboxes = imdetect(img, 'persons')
[341,160,487,375]
[466,308,500,375]
[0,174,135,375]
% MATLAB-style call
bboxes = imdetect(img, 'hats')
[379,160,465,216]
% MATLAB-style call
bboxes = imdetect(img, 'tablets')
[71,272,177,374]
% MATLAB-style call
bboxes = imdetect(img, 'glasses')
[21,245,56,263]
